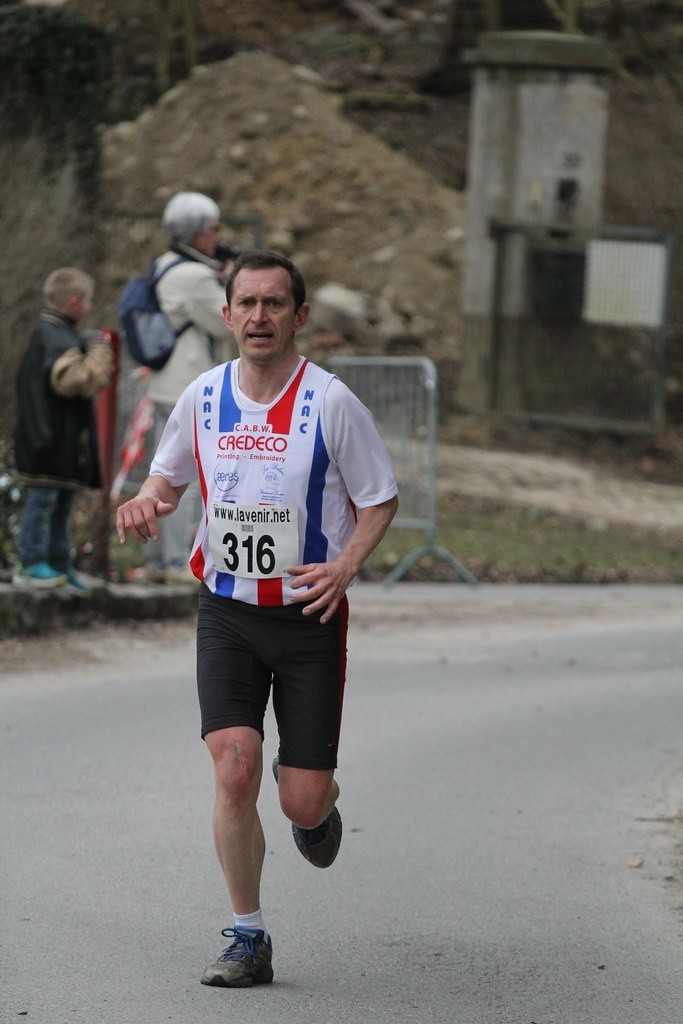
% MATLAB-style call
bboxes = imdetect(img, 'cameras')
[213,242,240,273]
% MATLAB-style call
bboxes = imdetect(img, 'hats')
[165,191,219,244]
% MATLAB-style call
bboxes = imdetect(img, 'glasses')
[210,223,223,233]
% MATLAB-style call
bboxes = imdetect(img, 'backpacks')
[118,253,197,371]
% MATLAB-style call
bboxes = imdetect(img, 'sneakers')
[272,756,342,868]
[200,926,274,988]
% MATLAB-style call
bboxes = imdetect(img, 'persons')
[116,250,398,989]
[13,267,113,595]
[147,192,233,586]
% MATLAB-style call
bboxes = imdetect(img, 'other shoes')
[52,562,93,596]
[12,561,67,587]
